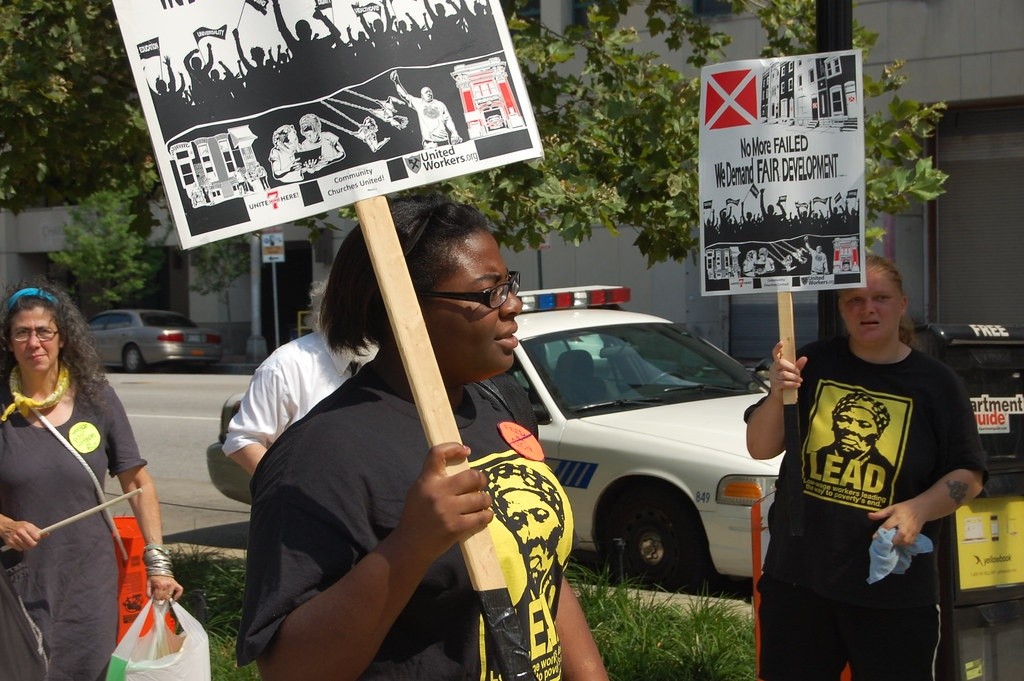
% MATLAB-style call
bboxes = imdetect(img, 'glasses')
[422,270,523,311]
[10,327,60,343]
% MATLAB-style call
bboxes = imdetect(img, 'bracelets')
[140,542,176,577]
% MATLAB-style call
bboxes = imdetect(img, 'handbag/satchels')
[108,591,211,680]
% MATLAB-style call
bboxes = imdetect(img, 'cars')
[221,285,787,593]
[84,308,224,371]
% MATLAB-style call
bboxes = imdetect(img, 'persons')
[743,256,989,681]
[221,285,379,476]
[229,190,607,681]
[0,282,182,681]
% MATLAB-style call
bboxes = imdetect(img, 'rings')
[168,592,173,596]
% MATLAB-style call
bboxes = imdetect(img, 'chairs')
[554,349,612,408]
